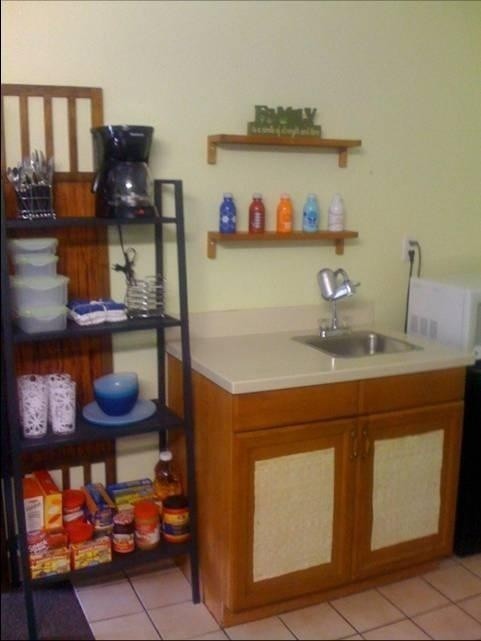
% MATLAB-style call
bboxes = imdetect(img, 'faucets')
[317,267,362,337]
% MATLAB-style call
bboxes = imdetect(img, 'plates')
[82,398,157,426]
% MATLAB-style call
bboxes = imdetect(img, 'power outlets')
[401,234,418,261]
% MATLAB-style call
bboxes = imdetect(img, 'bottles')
[154,451,182,499]
[302,195,320,233]
[328,193,344,232]
[249,192,264,234]
[276,193,293,233]
[219,193,237,234]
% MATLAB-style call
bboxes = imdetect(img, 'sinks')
[293,328,425,357]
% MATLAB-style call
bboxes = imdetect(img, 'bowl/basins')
[93,370,139,417]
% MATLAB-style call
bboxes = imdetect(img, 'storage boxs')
[1,175,202,636]
[4,235,74,337]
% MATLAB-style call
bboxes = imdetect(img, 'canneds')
[135,502,161,551]
[162,496,191,544]
[112,512,135,554]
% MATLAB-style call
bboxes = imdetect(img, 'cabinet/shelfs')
[207,134,360,258]
[164,354,469,627]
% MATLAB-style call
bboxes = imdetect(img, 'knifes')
[5,149,55,220]
